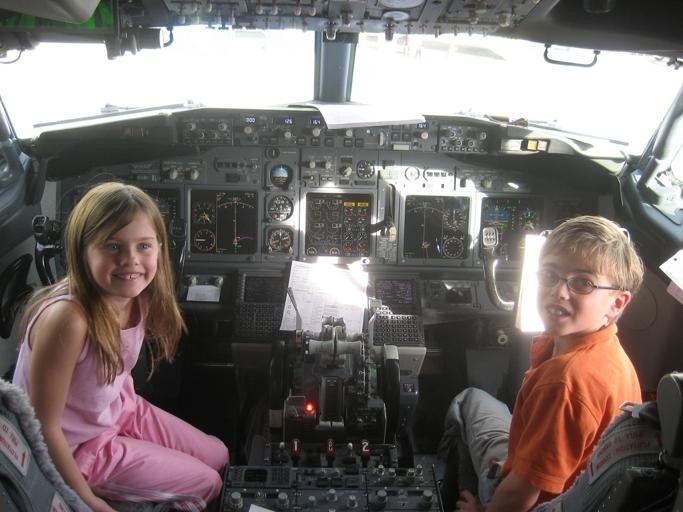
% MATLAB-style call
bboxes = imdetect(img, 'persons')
[8,180,231,512]
[440,215,645,512]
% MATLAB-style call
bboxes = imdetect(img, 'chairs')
[526,367,682,512]
[1,375,95,512]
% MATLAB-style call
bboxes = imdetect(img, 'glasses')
[537,268,619,296]
